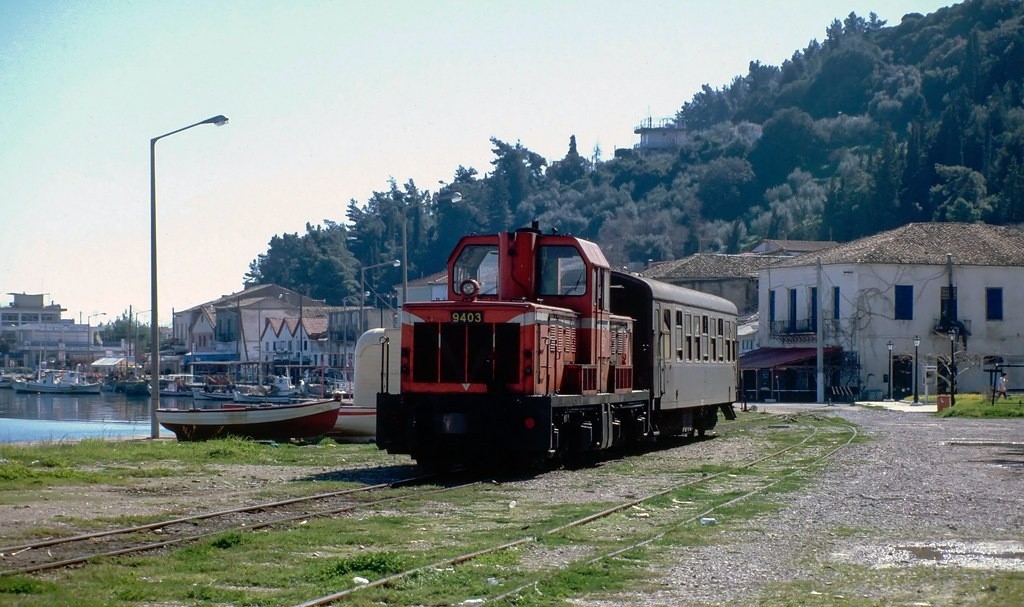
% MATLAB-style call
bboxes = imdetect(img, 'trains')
[354,219,741,483]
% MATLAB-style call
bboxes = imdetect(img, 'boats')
[0,288,376,437]
[154,385,342,444]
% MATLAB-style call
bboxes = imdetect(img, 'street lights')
[401,192,464,305]
[912,336,923,406]
[946,327,957,407]
[886,340,894,402]
[135,309,152,366]
[358,260,401,337]
[150,114,230,447]
[87,313,107,362]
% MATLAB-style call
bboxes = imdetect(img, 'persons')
[996,373,1009,401]
